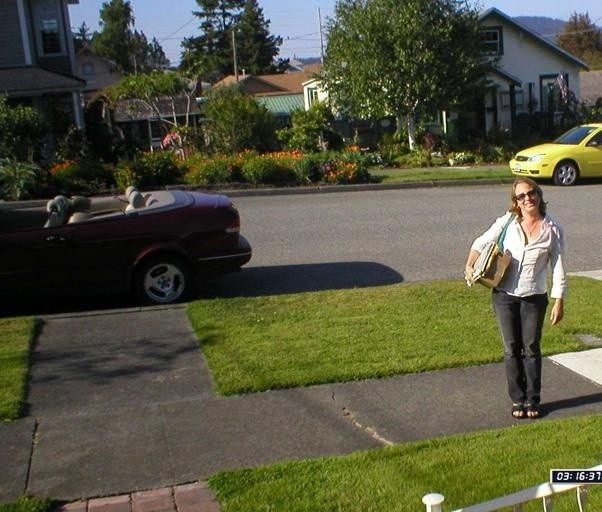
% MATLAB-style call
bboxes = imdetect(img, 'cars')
[509,121,601,187]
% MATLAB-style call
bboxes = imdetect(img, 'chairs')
[43,201,67,227]
[48,194,68,208]
[124,185,136,196]
[122,192,145,212]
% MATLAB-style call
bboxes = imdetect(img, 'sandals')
[523,405,543,419]
[511,404,525,419]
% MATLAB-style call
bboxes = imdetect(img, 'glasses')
[513,189,536,201]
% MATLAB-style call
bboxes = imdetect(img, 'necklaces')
[522,218,539,237]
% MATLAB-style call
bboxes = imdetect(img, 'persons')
[465,177,568,419]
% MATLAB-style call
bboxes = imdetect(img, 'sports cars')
[0,181,254,307]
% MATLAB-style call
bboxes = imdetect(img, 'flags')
[557,71,568,104]
